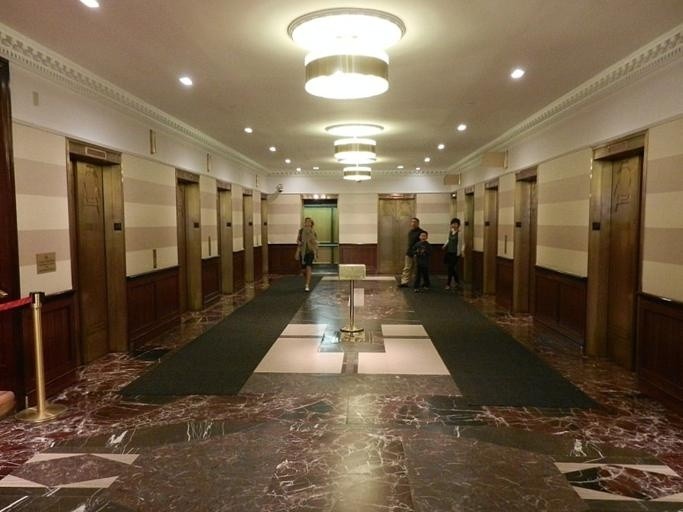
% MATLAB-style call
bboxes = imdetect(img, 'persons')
[296,217,316,292]
[441,216,464,291]
[412,230,432,295]
[299,221,320,276]
[394,217,423,289]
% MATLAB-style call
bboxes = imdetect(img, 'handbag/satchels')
[293,228,303,262]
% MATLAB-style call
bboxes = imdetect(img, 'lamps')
[284,3,406,185]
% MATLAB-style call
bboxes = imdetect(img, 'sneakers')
[396,283,462,294]
[303,285,310,292]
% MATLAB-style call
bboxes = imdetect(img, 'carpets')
[394,272,601,406]
[111,272,325,398]
